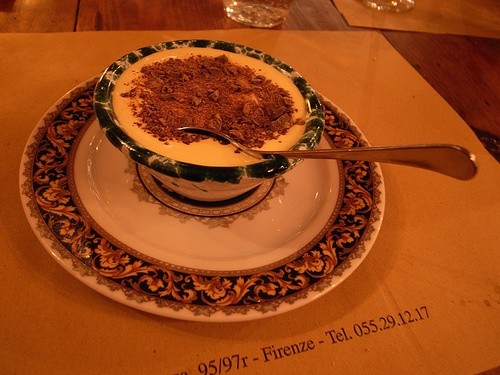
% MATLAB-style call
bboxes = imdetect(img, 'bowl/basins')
[94,38,326,201]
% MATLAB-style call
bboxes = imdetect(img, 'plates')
[19,72,386,322]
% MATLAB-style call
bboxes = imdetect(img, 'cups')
[223,0,295,27]
[361,0,414,13]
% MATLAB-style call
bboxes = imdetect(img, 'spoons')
[176,125,478,181]
